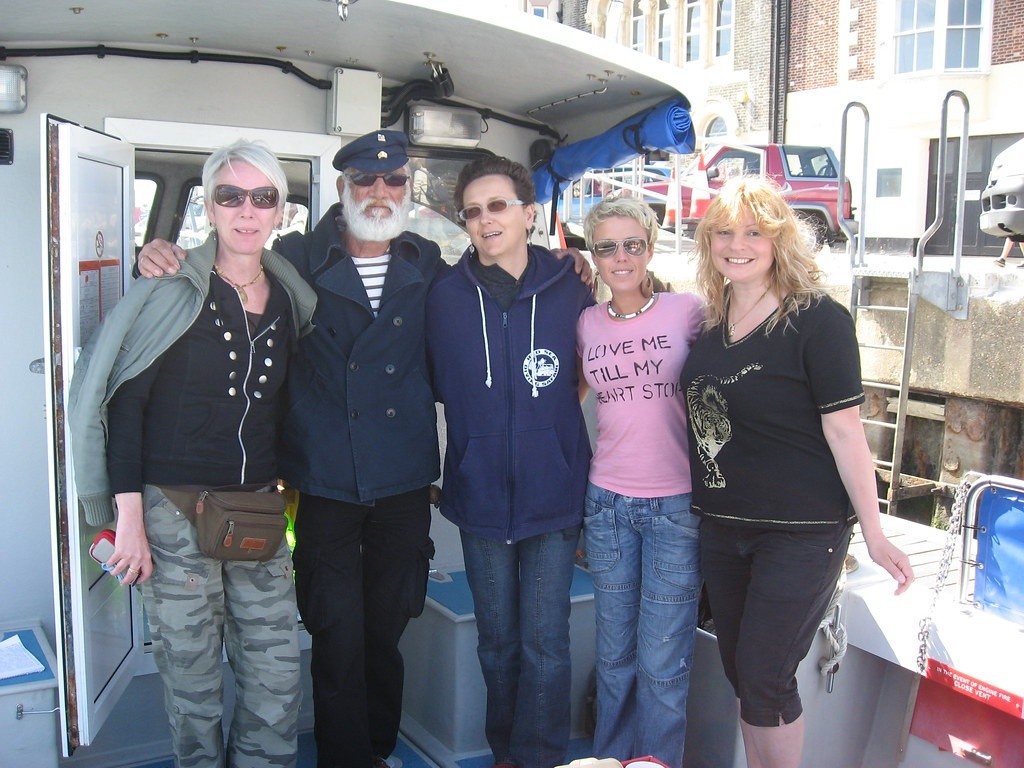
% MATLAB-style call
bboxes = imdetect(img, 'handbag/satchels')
[160,484,288,561]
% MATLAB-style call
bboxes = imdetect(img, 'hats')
[332,130,410,173]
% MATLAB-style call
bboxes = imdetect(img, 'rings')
[140,257,143,263]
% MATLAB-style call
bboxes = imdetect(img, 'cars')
[555,165,673,221]
[979,138,1024,238]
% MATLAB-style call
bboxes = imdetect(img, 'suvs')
[606,142,857,255]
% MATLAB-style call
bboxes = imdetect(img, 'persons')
[678,174,916,768]
[993,234,1024,269]
[67,136,318,768]
[425,158,598,768]
[134,130,594,768]
[575,193,708,768]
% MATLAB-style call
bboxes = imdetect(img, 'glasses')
[592,237,648,260]
[459,198,525,221]
[344,172,410,187]
[212,184,279,209]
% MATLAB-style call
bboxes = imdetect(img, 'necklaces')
[729,284,771,337]
[214,263,263,305]
[608,291,655,318]
